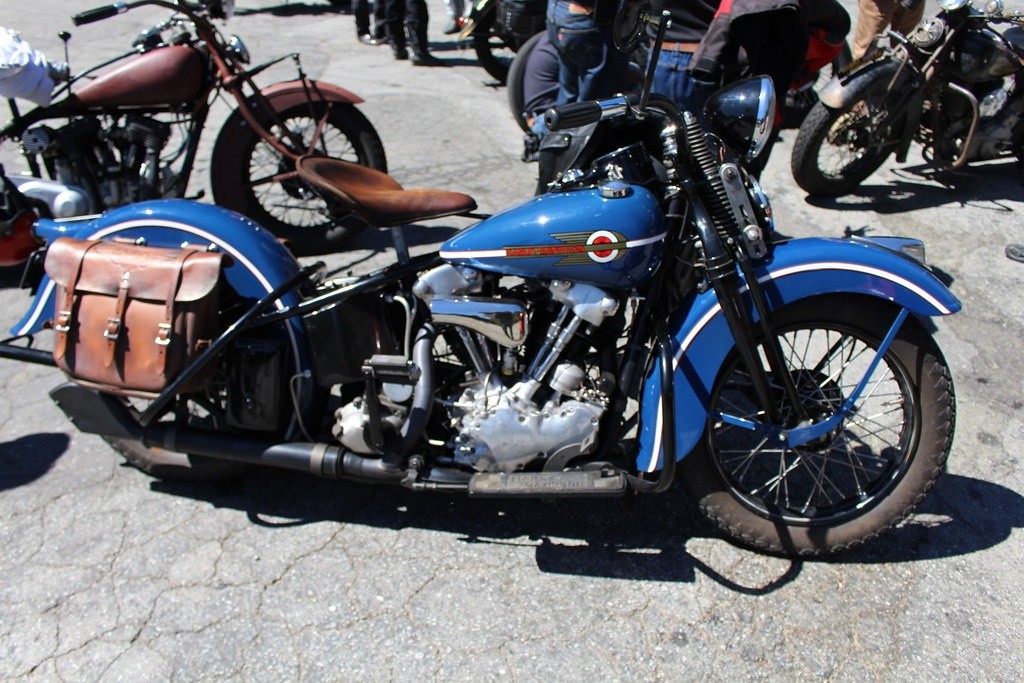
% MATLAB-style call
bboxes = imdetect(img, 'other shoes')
[1004,243,1024,264]
[395,51,408,60]
[358,34,374,43]
[445,21,460,34]
[370,36,388,45]
[412,53,443,66]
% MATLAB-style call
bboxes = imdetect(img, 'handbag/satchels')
[43,236,233,396]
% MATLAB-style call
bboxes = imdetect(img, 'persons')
[0,25,69,107]
[353,0,925,162]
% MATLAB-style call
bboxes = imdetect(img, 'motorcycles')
[789,0,1024,199]
[456,0,855,162]
[0,0,389,273]
[0,21,960,556]
